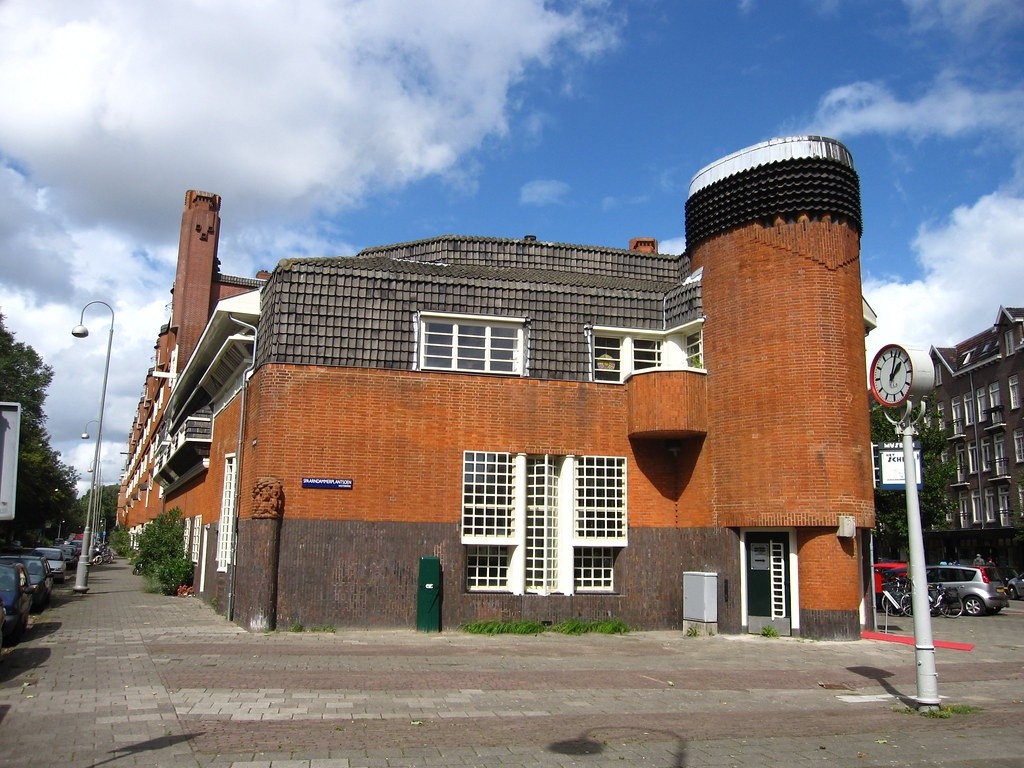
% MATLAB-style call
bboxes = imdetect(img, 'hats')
[976,554,981,556]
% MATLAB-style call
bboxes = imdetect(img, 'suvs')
[886,565,1008,614]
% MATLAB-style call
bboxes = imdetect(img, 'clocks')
[874,347,912,403]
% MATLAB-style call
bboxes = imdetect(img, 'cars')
[0,534,100,648]
[1007,572,1024,600]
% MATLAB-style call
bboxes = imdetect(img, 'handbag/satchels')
[944,587,960,602]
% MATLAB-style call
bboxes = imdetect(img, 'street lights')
[88,459,101,546]
[57,518,66,539]
[81,419,103,563]
[69,300,116,594]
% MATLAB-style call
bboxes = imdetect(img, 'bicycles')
[882,574,963,619]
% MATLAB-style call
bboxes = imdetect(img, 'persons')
[940,558,960,567]
[972,553,1008,566]
